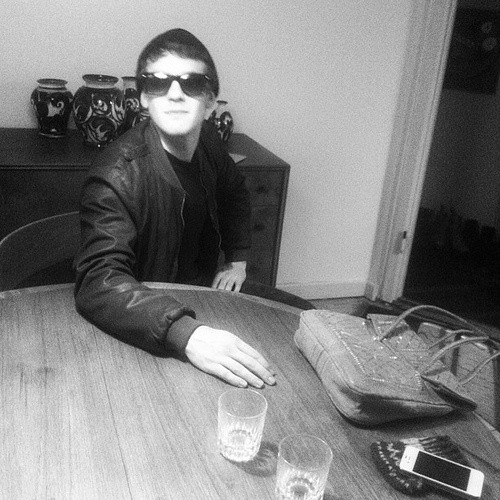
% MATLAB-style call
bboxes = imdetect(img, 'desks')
[0,282,500,500]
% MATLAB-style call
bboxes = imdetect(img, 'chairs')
[414,322,499,429]
[0,212,83,293]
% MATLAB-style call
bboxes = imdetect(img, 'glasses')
[141,71,211,100]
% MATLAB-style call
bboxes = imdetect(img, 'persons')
[73,28,317,390]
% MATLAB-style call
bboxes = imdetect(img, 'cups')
[218,389,268,462]
[274,434,333,500]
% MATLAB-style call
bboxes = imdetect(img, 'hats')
[136,27,219,97]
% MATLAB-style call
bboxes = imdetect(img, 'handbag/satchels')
[293,303,500,428]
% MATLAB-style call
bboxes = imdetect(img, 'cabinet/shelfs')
[0,127,291,289]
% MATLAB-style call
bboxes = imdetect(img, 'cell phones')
[399,445,484,500]
